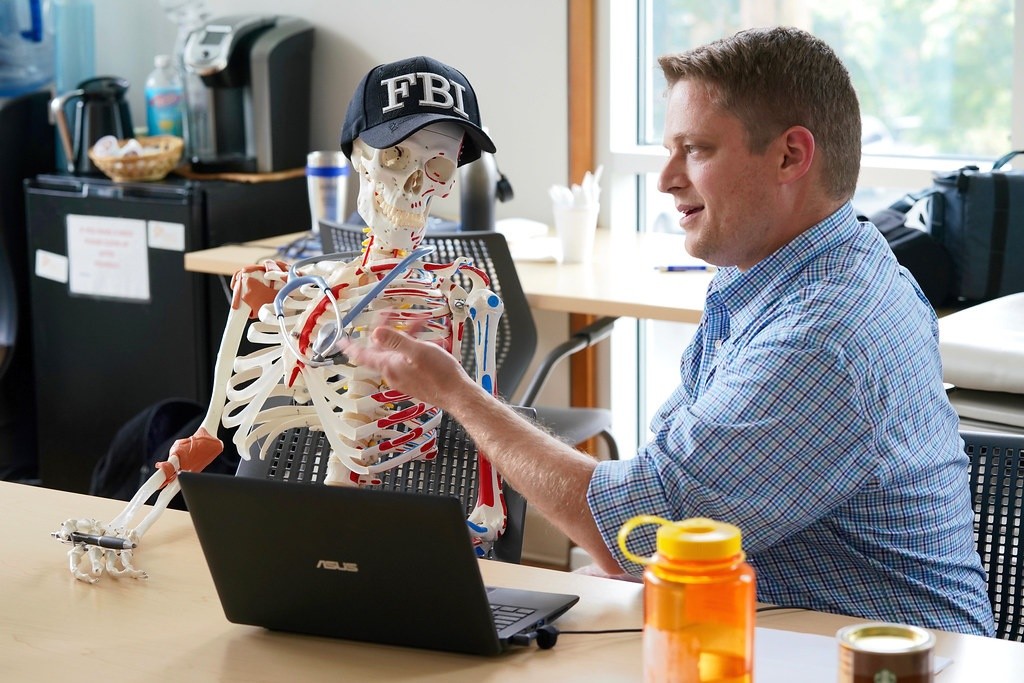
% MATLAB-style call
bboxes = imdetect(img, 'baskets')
[88,135,183,183]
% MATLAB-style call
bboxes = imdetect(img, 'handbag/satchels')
[860,209,950,313]
[928,150,1024,305]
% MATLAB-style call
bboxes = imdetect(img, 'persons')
[335,27,997,637]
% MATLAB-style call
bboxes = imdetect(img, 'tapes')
[834,618,936,683]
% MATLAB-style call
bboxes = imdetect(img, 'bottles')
[616,516,758,683]
[144,56,183,139]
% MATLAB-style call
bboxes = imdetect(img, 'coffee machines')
[174,14,315,173]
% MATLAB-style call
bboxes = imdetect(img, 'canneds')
[837,622,936,683]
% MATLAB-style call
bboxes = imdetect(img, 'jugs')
[53,76,135,176]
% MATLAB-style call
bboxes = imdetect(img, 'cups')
[307,151,349,231]
[552,203,600,261]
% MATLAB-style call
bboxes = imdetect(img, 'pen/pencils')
[655,265,717,272]
[50,531,137,550]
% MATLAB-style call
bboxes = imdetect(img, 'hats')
[340,56,496,168]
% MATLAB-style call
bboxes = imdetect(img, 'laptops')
[178,471,580,653]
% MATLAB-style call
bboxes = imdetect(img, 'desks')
[0,481,1024,683]
[181,224,1023,431]
